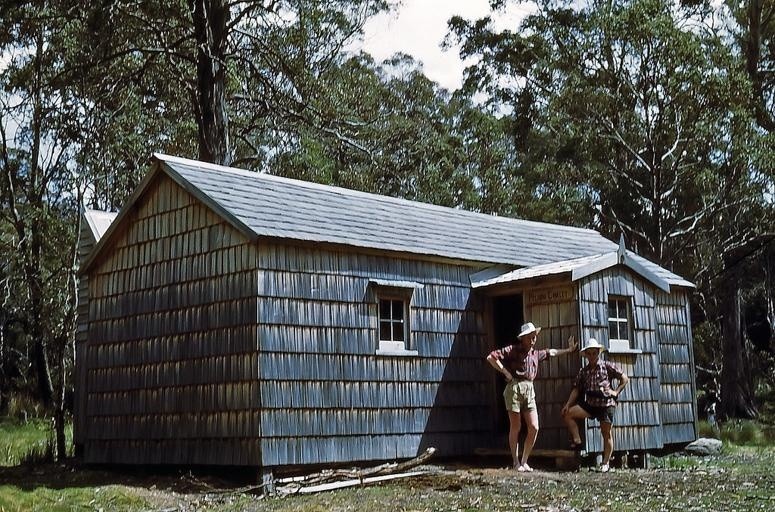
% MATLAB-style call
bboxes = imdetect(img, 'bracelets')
[567,348,570,353]
[500,367,506,372]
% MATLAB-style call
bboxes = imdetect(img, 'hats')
[579,338,604,357]
[518,322,542,337]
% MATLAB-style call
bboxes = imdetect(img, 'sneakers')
[513,464,533,472]
[564,441,583,451]
[595,463,610,473]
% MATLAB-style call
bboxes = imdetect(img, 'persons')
[487,322,578,472]
[561,338,628,472]
